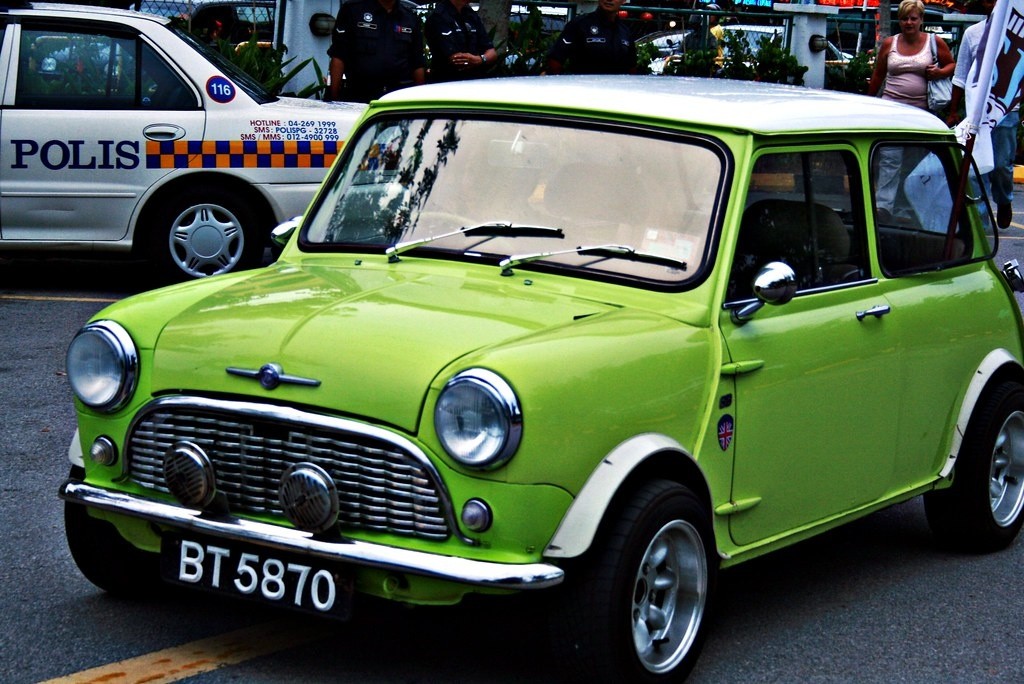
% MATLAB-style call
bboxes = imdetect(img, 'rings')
[465,62,468,65]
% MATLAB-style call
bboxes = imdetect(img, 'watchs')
[481,54,486,63]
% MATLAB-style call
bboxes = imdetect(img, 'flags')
[904,0,1024,236]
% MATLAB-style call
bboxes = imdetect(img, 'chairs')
[736,199,850,265]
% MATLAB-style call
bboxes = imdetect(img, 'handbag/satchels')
[927,33,953,109]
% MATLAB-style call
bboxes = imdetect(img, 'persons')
[327,0,426,103]
[546,0,638,75]
[425,0,498,82]
[864,0,955,224]
[672,3,726,77]
[948,0,1019,238]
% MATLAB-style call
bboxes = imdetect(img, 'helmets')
[705,4,722,25]
[690,9,709,32]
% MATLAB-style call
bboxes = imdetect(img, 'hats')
[208,20,222,32]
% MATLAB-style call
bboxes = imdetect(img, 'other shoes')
[877,208,890,215]
[997,202,1012,229]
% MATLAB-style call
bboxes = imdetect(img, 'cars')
[56,74,1024,684]
[633,28,694,63]
[649,23,855,82]
[129,0,278,53]
[0,0,411,291]
